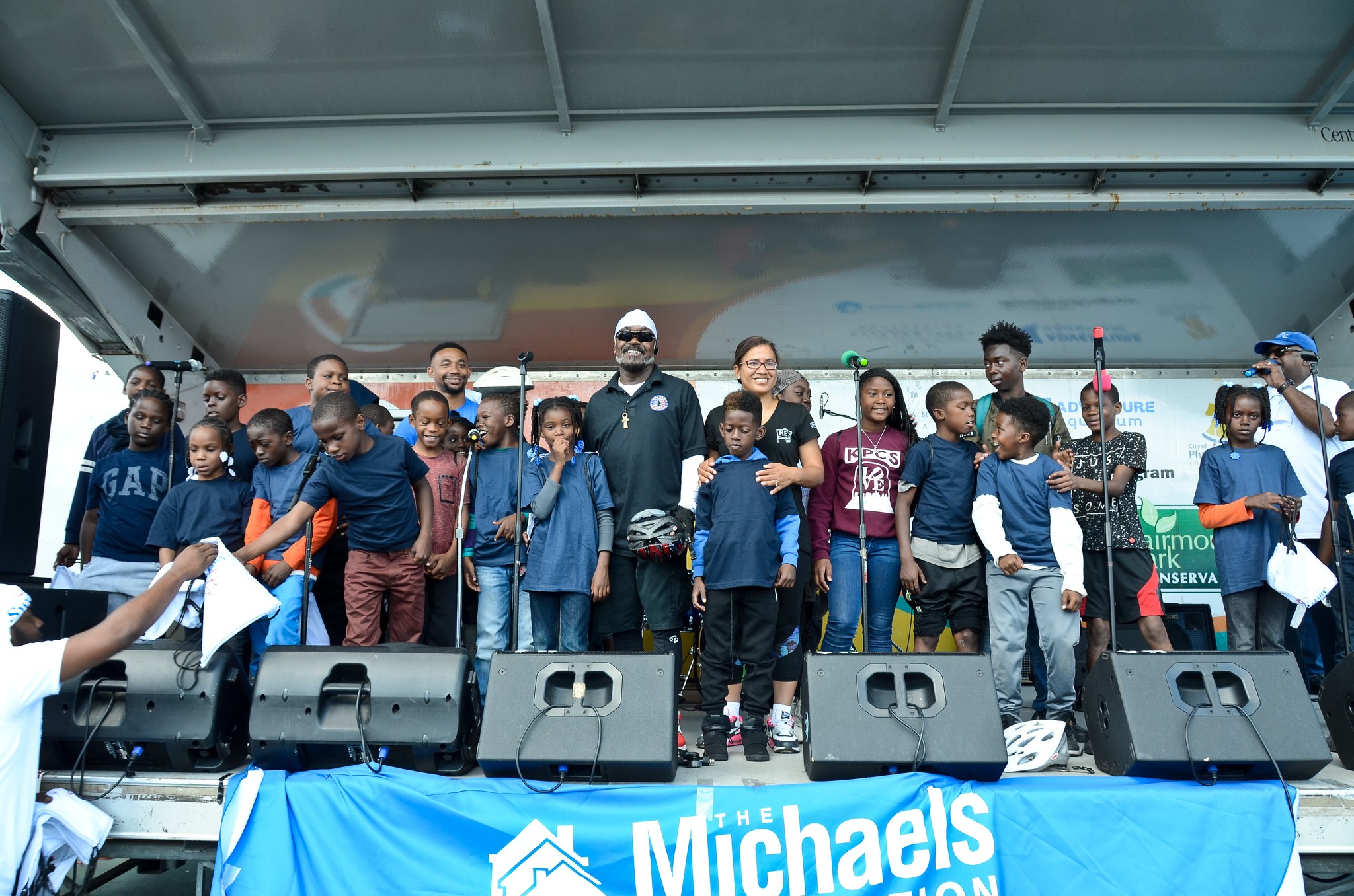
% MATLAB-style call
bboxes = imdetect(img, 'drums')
[643,610,694,633]
[699,619,708,657]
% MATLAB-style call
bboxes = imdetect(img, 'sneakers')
[1064,728,1080,755]
[789,703,804,742]
[765,704,800,753]
[696,708,743,748]
[676,710,687,751]
[701,715,732,761]
[1001,715,1021,731]
[740,715,769,761]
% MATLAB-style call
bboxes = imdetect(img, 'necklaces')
[618,388,636,429]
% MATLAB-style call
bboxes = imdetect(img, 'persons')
[75,390,186,598]
[695,335,825,753]
[807,368,923,653]
[1192,384,1307,646]
[1253,332,1354,695]
[391,342,480,458]
[51,323,1354,769]
[0,541,220,896]
[972,398,1087,758]
[690,390,801,761]
[231,391,434,647]
[581,310,709,751]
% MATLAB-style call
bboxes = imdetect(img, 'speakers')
[1083,650,1332,777]
[39,650,250,772]
[0,581,110,640]
[478,649,680,782]
[797,650,1008,780]
[0,289,62,577]
[243,643,476,775]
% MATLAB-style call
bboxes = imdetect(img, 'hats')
[614,308,658,345]
[772,370,807,398]
[1254,331,1317,355]
[1,583,31,630]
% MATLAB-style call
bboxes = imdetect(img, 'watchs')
[1277,378,1296,394]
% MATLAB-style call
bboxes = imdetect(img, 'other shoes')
[1307,676,1325,699]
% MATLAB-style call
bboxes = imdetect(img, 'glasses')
[615,330,655,343]
[739,361,778,370]
[1261,347,1302,360]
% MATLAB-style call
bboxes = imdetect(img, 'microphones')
[1245,360,1278,377]
[147,360,202,372]
[820,394,823,419]
[842,351,868,368]
[1092,327,1111,393]
[468,429,481,443]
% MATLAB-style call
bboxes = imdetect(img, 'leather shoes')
[1071,719,1090,742]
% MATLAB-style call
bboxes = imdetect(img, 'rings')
[774,479,779,487]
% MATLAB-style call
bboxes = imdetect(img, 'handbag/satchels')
[1268,496,1338,609]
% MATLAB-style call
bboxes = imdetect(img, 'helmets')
[1001,719,1070,772]
[473,367,534,391]
[626,510,687,558]
